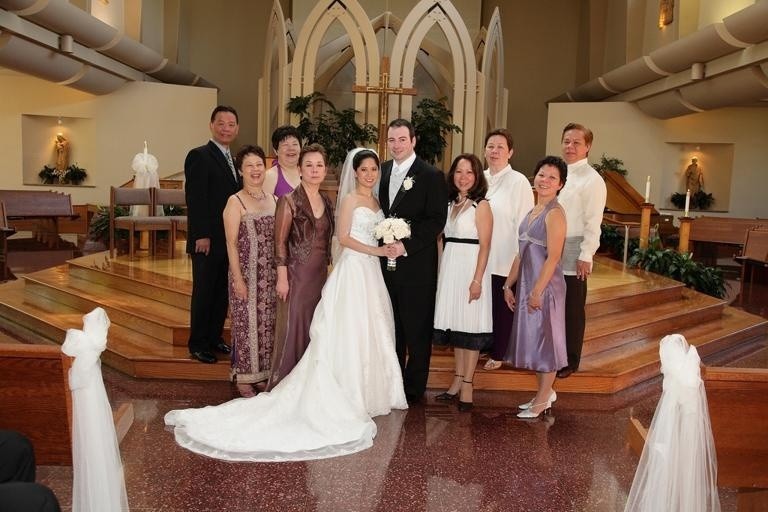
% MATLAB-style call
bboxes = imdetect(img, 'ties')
[226,152,234,171]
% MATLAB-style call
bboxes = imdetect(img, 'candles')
[646,176,651,203]
[685,189,691,217]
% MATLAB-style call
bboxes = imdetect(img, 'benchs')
[0,342,134,467]
[628,366,768,511]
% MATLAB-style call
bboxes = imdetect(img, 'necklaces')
[244,187,267,201]
[366,196,375,201]
[451,197,467,207]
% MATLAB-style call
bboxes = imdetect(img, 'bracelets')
[368,246,371,258]
[529,291,536,297]
[502,285,511,290]
[472,279,482,287]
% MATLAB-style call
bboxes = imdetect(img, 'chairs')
[153,186,187,258]
[110,185,172,261]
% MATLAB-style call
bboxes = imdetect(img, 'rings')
[584,270,589,275]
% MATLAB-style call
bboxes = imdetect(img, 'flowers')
[371,212,412,272]
[401,175,416,193]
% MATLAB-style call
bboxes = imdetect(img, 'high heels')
[233,378,256,398]
[253,381,266,393]
[482,359,502,370]
[517,399,552,418]
[518,391,557,410]
[458,380,473,411]
[435,375,464,400]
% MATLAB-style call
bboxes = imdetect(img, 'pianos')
[602,171,673,249]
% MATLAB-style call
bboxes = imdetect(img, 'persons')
[478,127,535,370]
[377,118,448,405]
[223,145,280,398]
[685,156,705,198]
[434,153,493,413]
[54,133,70,171]
[503,156,567,419]
[0,429,62,512]
[164,147,410,463]
[260,124,303,199]
[264,143,335,392]
[184,105,244,366]
[556,123,608,379]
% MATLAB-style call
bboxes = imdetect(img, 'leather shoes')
[217,341,231,354]
[191,351,217,364]
[556,367,574,378]
